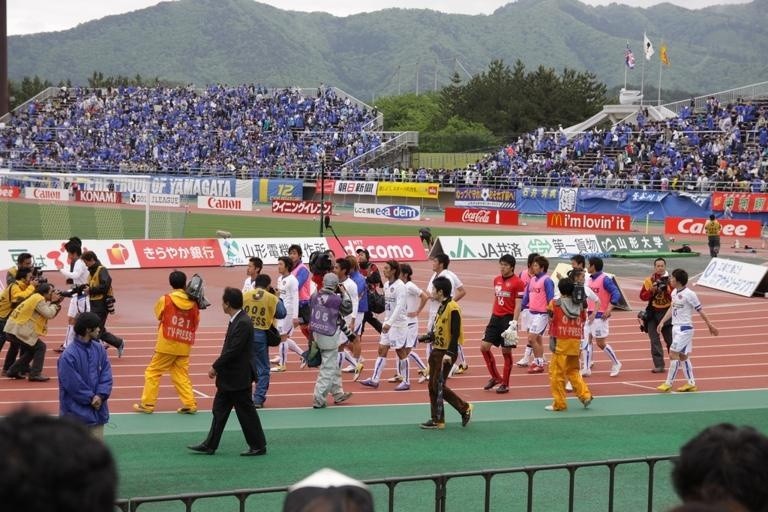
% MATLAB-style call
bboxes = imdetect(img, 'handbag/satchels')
[301,341,322,367]
[643,306,656,321]
[266,325,280,347]
[367,288,384,314]
[297,298,310,323]
[548,335,557,352]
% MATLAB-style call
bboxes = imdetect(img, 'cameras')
[657,281,667,292]
[33,266,43,276]
[104,296,116,315]
[267,286,280,294]
[57,290,73,298]
[32,277,47,286]
[416,332,434,344]
[71,283,89,296]
[335,283,344,294]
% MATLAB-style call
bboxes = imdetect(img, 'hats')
[356,248,369,256]
[323,273,339,290]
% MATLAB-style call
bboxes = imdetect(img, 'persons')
[133,271,197,413]
[0,410,116,512]
[418,276,472,429]
[283,468,375,510]
[640,258,675,372]
[657,268,719,392]
[309,273,353,407]
[2,237,124,381]
[187,286,267,457]
[481,254,525,393]
[517,253,622,376]
[704,214,722,258]
[419,228,433,249]
[547,278,592,411]
[243,245,467,391]
[725,204,733,218]
[0,82,768,192]
[673,424,768,511]
[57,313,114,438]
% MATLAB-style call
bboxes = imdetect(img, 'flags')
[661,46,670,65]
[644,34,656,61]
[625,45,635,70]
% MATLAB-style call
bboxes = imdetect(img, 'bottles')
[734,238,740,248]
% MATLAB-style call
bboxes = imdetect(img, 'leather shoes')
[186,440,218,456]
[240,444,267,457]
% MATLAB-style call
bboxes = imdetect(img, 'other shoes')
[270,364,287,372]
[447,361,469,377]
[334,391,353,404]
[313,402,332,409]
[271,357,281,364]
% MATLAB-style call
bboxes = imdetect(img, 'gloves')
[500,320,519,347]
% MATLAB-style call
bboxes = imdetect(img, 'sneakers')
[341,363,430,390]
[2,341,125,382]
[419,418,447,430]
[582,400,593,409]
[131,402,154,416]
[483,356,621,394]
[174,403,198,416]
[544,404,566,412]
[459,401,471,427]
[651,366,696,392]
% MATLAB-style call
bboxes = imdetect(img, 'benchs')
[483,96,768,192]
